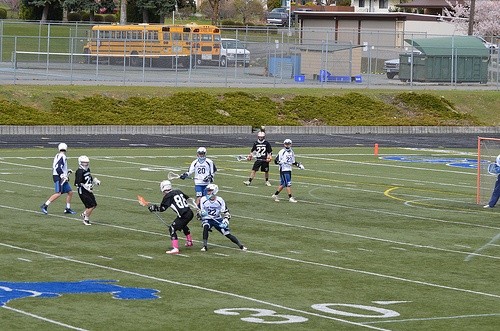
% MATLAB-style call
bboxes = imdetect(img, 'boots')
[185,234,193,246]
[166,240,179,254]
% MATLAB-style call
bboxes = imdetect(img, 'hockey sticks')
[186,198,222,224]
[60,169,73,186]
[487,161,500,177]
[137,195,170,227]
[237,155,267,161]
[167,171,199,181]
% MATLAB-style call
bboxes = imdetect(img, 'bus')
[81,22,221,69]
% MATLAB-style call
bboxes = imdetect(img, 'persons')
[272,139,304,203]
[483,154,500,209]
[196,184,247,251]
[40,143,78,214]
[74,155,101,226]
[180,147,218,221]
[148,180,194,254]
[243,132,273,187]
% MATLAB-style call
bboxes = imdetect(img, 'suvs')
[221,37,250,67]
[266,6,291,28]
[383,51,424,79]
[472,35,499,54]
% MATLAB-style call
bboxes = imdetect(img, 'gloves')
[67,169,73,173]
[279,160,287,165]
[84,184,94,192]
[148,204,160,212]
[203,174,214,183]
[222,218,229,228]
[60,174,68,183]
[180,172,188,180]
[198,209,208,217]
[93,178,100,186]
[299,164,304,170]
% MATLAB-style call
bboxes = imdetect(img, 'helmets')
[197,147,207,159]
[160,180,172,192]
[205,184,219,199]
[284,139,292,151]
[78,155,90,171]
[258,132,266,143]
[58,143,67,152]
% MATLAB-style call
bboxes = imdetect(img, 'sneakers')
[272,194,280,202]
[243,180,251,186]
[80,213,86,219]
[63,208,77,215]
[201,247,209,252]
[484,205,495,208]
[289,197,297,203]
[83,219,91,226]
[265,181,272,187]
[40,203,48,215]
[241,247,247,251]
